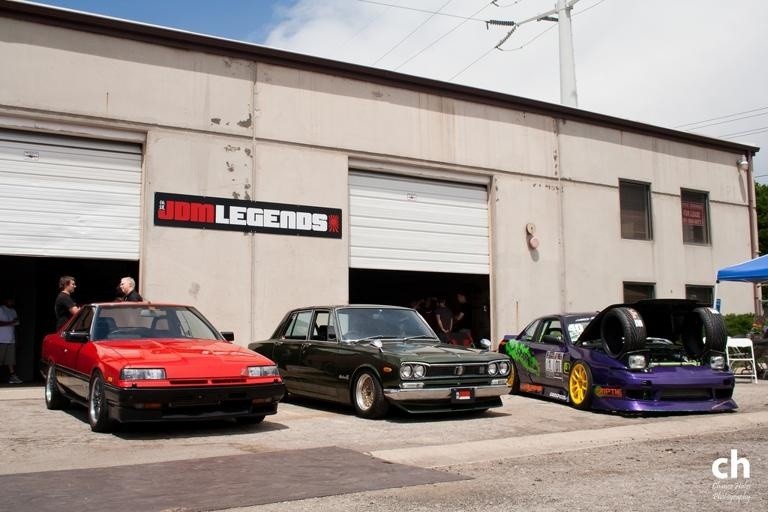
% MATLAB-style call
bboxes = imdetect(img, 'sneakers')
[9,375,23,383]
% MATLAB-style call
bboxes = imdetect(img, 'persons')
[454,291,472,346]
[413,297,439,331]
[433,295,453,343]
[0,294,24,384]
[54,275,80,379]
[119,276,142,301]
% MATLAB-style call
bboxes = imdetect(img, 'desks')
[751,338,768,379]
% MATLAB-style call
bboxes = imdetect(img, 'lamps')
[737,154,749,169]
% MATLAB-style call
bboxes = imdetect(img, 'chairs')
[88,317,117,341]
[725,334,757,384]
[319,325,338,341]
